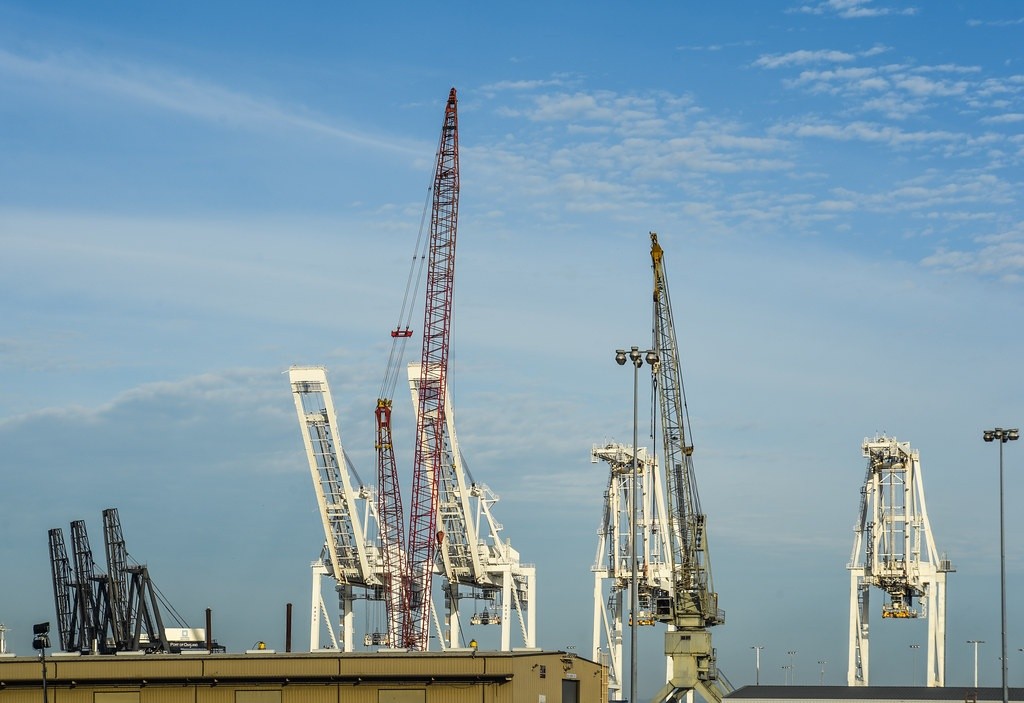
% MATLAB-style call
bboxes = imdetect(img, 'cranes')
[376,87,460,651]
[649,230,735,703]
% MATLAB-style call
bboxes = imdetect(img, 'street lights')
[751,646,763,686]
[818,662,828,686]
[781,651,797,686]
[615,346,660,703]
[966,641,984,688]
[985,428,1020,703]
[910,645,919,686]
